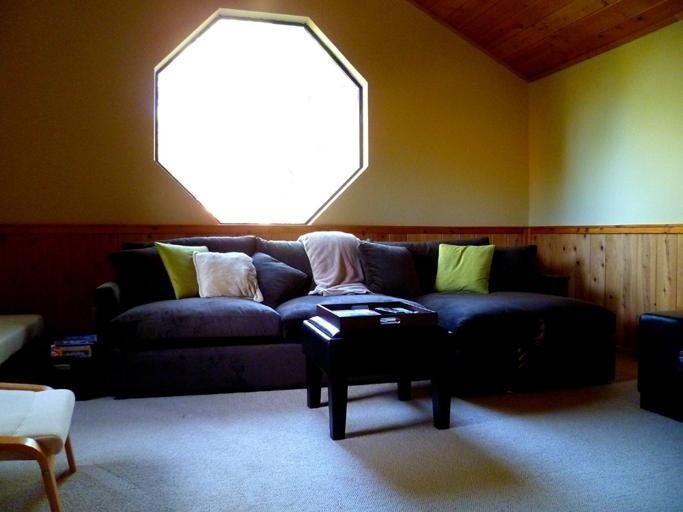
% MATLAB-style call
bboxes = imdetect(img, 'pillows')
[433,239,495,295]
[434,236,489,277]
[357,244,425,300]
[173,235,256,259]
[153,239,209,301]
[107,241,176,315]
[190,247,264,304]
[255,238,314,291]
[488,245,540,292]
[252,251,306,307]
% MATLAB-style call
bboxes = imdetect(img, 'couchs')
[98,234,618,397]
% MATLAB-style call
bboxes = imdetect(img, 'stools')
[0,382,76,511]
[0,313,45,383]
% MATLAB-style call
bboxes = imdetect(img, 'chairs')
[636,309,683,422]
